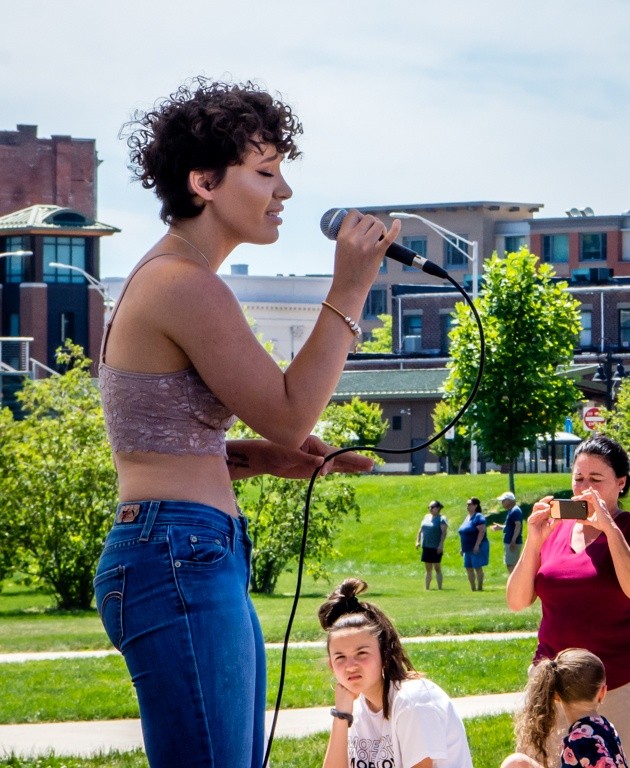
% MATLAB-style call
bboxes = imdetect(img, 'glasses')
[466,503,474,506]
[428,505,436,509]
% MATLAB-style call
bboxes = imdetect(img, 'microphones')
[320,207,449,280]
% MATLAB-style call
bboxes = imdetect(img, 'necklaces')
[577,524,598,540]
[165,234,211,269]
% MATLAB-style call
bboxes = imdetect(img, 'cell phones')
[549,499,588,520]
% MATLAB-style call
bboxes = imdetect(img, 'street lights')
[48,262,112,322]
[389,210,481,475]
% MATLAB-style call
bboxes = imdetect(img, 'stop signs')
[583,406,607,431]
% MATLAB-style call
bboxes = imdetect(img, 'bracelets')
[330,709,353,728]
[322,301,361,355]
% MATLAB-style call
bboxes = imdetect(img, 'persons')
[318,579,473,768]
[499,647,630,768]
[95,80,401,768]
[490,492,523,574]
[457,498,489,591]
[506,436,630,768]
[416,501,449,589]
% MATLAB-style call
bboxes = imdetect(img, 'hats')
[496,492,515,501]
